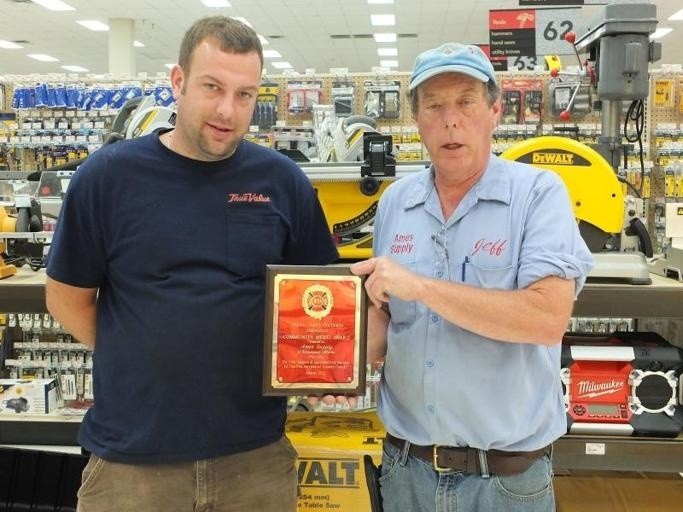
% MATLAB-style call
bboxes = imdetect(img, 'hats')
[408,42,498,95]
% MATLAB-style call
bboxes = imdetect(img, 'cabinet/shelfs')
[0,190,95,456]
[244,75,428,162]
[493,75,682,250]
[547,277,683,474]
[0,76,172,191]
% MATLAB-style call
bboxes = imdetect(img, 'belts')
[386,433,552,475]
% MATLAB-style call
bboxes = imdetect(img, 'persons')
[46,15,338,512]
[305,41,592,511]
